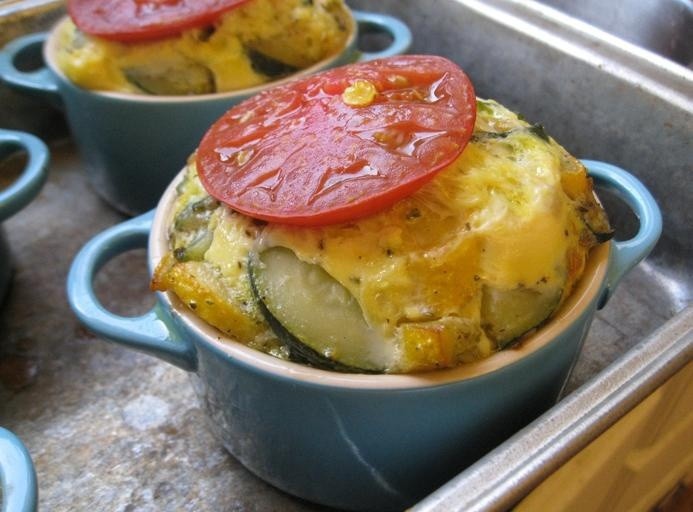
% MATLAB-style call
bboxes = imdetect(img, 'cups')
[3,12,408,217]
[67,158,668,510]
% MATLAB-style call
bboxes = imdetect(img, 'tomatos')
[152,53,489,225]
[63,1,239,41]
